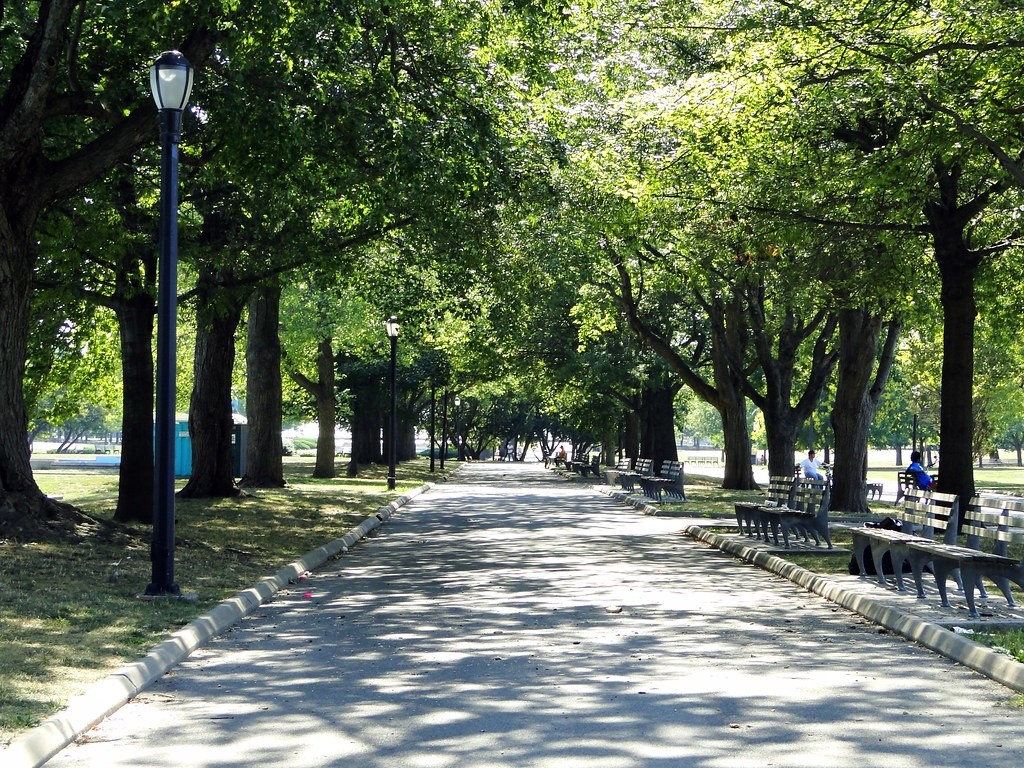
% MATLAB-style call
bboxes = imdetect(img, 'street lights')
[145,50,195,597]
[455,394,460,460]
[913,384,920,452]
[385,315,401,491]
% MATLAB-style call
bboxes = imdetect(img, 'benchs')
[757,478,833,550]
[894,472,938,506]
[625,459,654,492]
[684,456,718,466]
[641,459,671,498]
[847,487,964,592]
[618,457,645,490]
[905,497,1024,619]
[866,483,884,500]
[649,461,687,502]
[734,476,793,541]
[603,458,631,487]
[556,452,601,479]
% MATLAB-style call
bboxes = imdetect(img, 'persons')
[905,451,937,491]
[760,455,767,465]
[801,449,823,489]
[498,441,521,462]
[554,445,566,467]
